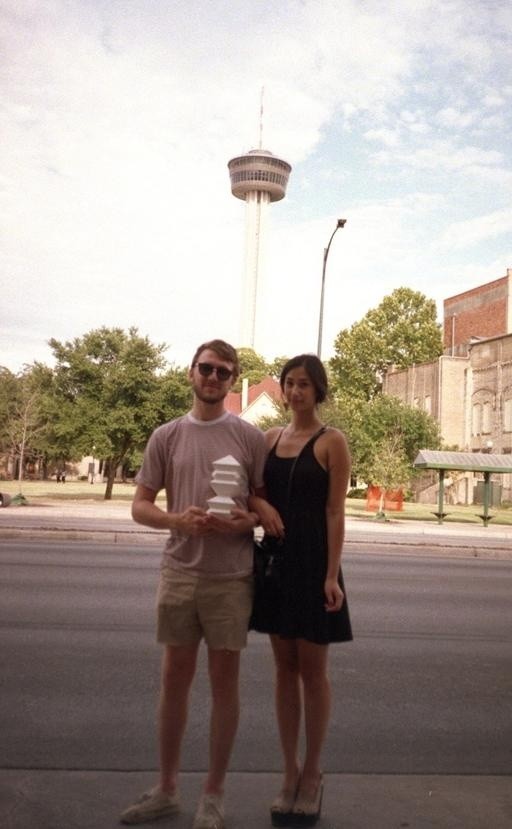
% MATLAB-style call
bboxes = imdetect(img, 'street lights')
[318,218,347,362]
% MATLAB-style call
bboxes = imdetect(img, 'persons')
[112,339,268,827]
[246,353,357,822]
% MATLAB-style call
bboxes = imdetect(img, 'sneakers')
[119,785,182,825]
[193,794,222,829]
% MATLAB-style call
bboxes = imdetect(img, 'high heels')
[269,771,326,819]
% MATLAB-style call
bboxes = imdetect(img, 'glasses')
[191,363,235,380]
